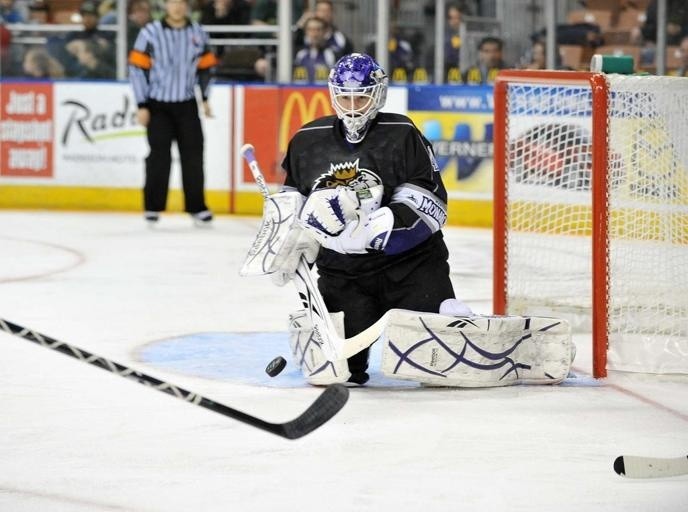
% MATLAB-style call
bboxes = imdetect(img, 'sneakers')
[188,210,213,221]
[144,209,160,221]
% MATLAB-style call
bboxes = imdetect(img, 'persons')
[126,0,215,223]
[241,52,574,387]
[1,1,687,83]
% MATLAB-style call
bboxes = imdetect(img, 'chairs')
[561,0,688,74]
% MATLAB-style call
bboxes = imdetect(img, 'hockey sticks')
[0,320,349,439]
[242,145,384,362]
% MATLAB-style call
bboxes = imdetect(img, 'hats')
[79,3,99,17]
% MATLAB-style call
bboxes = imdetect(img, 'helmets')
[328,53,388,143]
